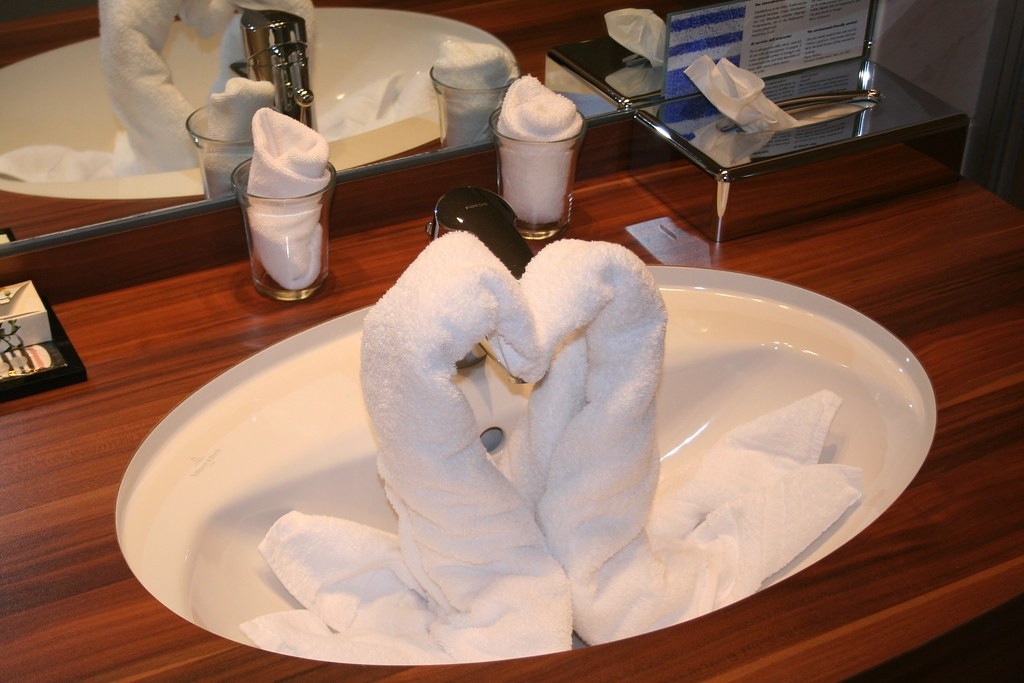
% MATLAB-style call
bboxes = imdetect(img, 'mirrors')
[0,0,727,259]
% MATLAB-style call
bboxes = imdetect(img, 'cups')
[186,106,255,198]
[229,158,340,300]
[429,66,521,147]
[488,108,585,240]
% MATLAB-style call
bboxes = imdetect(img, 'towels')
[495,74,583,226]
[237,228,861,667]
[242,108,330,289]
[197,75,277,198]
[0,2,431,189]
[436,39,513,146]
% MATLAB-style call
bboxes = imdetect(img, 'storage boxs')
[0,279,52,354]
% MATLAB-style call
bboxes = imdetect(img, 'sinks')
[113,264,948,668]
[3,4,520,202]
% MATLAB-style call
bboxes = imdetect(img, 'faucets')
[240,6,319,132]
[425,188,534,370]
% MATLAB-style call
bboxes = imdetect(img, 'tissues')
[624,52,971,242]
[543,6,667,118]
